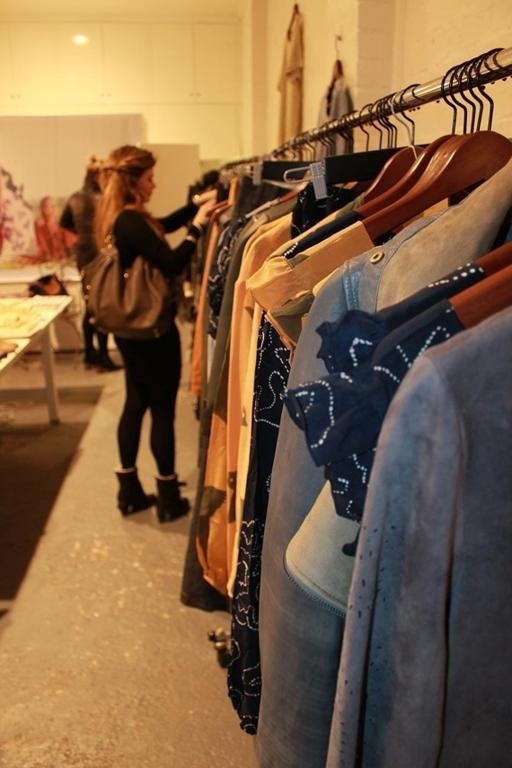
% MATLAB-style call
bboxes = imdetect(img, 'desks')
[0,294,83,425]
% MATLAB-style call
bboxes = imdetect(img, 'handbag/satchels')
[81,230,178,340]
[28,274,67,297]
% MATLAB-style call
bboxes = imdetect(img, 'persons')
[29,195,64,265]
[59,155,125,374]
[94,143,228,524]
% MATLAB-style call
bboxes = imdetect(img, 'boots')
[115,466,156,516]
[157,473,190,522]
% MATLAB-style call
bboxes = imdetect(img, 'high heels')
[84,351,122,372]
